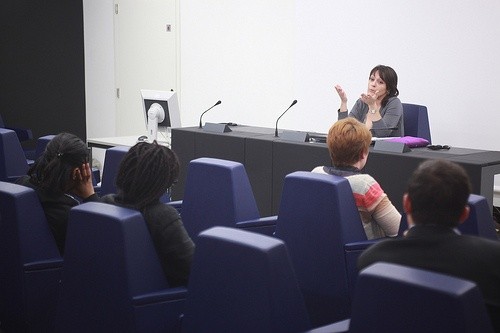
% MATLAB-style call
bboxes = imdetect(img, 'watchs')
[370,109,378,114]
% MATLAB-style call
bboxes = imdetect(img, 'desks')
[171,123,500,218]
[87,135,175,150]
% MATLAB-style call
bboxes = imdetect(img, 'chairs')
[0,127,495,333]
[402,100,433,143]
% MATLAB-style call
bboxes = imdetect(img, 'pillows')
[384,134,431,148]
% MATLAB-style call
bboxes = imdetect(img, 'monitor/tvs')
[140,89,181,143]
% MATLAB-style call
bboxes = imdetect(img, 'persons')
[334,65,404,137]
[349,160,500,333]
[103,142,195,286]
[16,132,99,260]
[310,117,401,241]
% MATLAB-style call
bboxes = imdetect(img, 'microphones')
[200,100,222,128]
[275,100,297,137]
